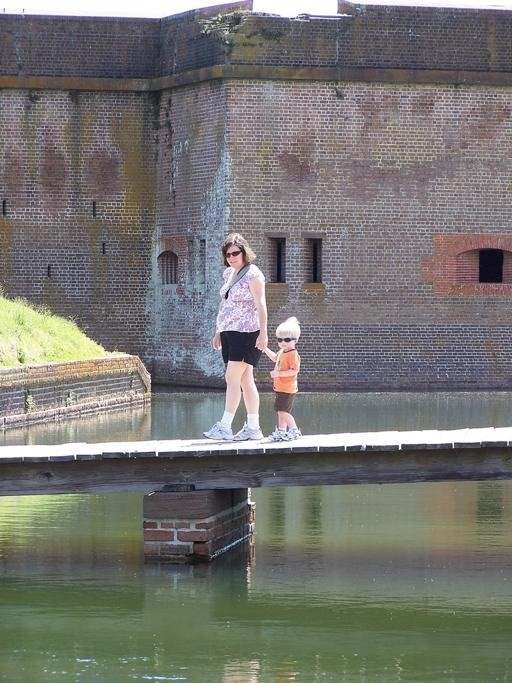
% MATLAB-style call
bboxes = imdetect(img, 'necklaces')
[220,267,249,300]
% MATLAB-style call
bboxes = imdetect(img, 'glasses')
[226,251,242,257]
[278,338,296,342]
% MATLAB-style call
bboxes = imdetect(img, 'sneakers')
[203,422,234,440]
[233,425,263,441]
[269,425,301,442]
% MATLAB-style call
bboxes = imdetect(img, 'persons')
[203,233,268,441]
[264,316,302,442]
